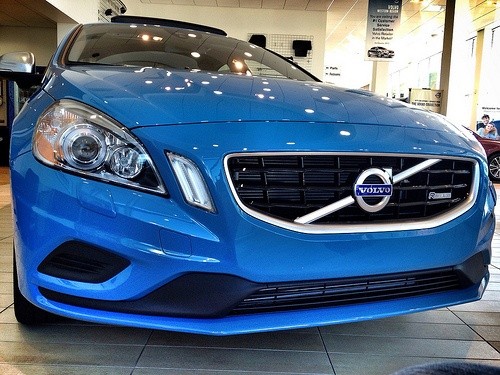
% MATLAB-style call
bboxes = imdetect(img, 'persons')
[475,124,498,140]
[476,115,495,131]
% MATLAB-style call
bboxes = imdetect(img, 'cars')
[460,125,500,183]
[367,46,395,58]
[0,15,498,336]
